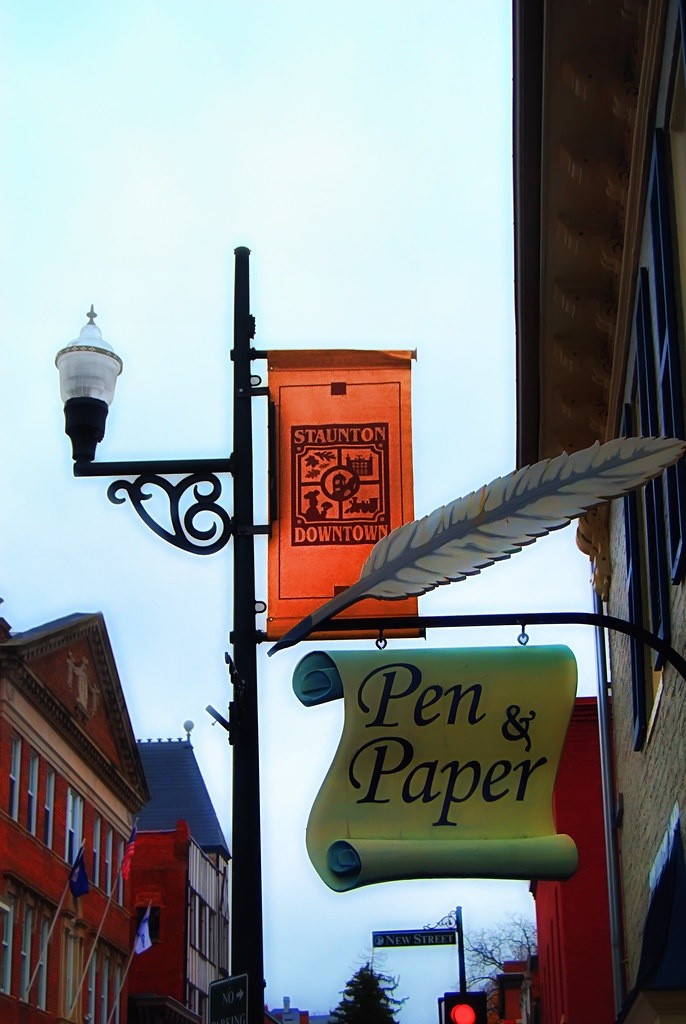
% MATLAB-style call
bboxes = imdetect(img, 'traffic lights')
[438,992,488,1024]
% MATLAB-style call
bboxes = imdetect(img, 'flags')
[120,823,134,881]
[65,844,89,897]
[131,905,152,954]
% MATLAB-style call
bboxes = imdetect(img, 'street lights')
[54,246,268,1023]
